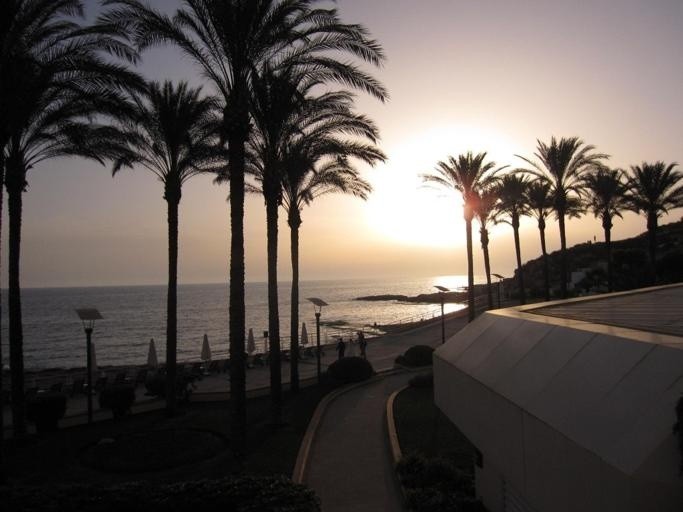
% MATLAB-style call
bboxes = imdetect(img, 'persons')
[335,338,346,360]
[355,330,368,359]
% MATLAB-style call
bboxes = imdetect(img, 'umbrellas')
[90,343,97,395]
[147,338,158,369]
[200,334,211,375]
[247,328,256,353]
[300,322,308,347]
